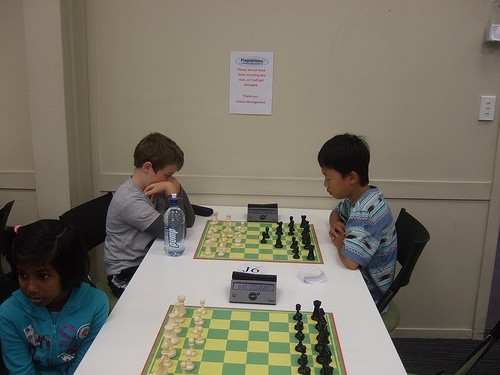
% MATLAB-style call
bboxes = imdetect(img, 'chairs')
[58,192,118,313]
[376,208,430,333]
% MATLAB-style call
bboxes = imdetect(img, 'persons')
[103,131,195,298]
[318,132,397,316]
[0,219,109,375]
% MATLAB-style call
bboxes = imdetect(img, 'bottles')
[163,193,185,257]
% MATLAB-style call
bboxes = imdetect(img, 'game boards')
[138,301,348,374]
[191,218,323,265]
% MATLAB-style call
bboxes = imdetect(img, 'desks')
[73,205,408,375]
